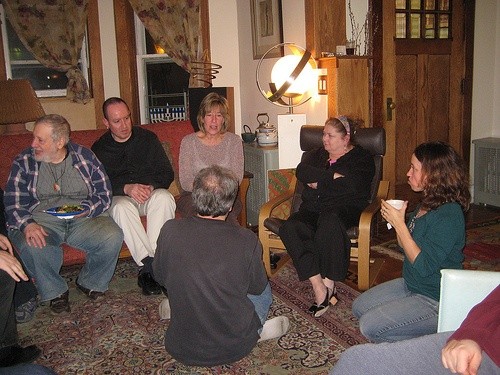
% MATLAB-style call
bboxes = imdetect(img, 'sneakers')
[15,296,38,323]
[75,277,105,303]
[0,344,41,367]
[49,290,71,316]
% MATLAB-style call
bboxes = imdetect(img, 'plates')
[42,206,88,219]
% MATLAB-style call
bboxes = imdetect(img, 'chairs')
[258,125,391,290]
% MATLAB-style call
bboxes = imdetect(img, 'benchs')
[0,121,254,267]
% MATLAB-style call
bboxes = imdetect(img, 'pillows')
[162,142,184,200]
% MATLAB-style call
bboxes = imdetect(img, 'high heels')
[313,288,338,317]
[308,285,337,313]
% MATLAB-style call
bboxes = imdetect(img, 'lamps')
[256,43,319,114]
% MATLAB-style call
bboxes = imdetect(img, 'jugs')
[255,124,278,147]
[241,125,255,144]
[253,112,274,143]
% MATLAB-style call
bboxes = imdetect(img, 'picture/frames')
[250,0,284,59]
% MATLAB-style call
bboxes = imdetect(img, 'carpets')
[371,215,500,272]
[11,248,373,375]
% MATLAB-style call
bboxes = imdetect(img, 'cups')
[384,199,404,212]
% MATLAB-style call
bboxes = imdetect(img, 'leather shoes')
[138,256,162,296]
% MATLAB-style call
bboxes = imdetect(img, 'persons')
[279,115,373,318]
[174,93,244,227]
[90,97,176,296]
[329,285,500,375]
[150,167,289,367]
[0,234,54,375]
[353,141,470,342]
[3,115,124,316]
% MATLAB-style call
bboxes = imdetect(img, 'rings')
[29,237,33,240]
[383,212,386,215]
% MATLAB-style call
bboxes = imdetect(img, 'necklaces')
[49,160,66,192]
[417,209,422,216]
